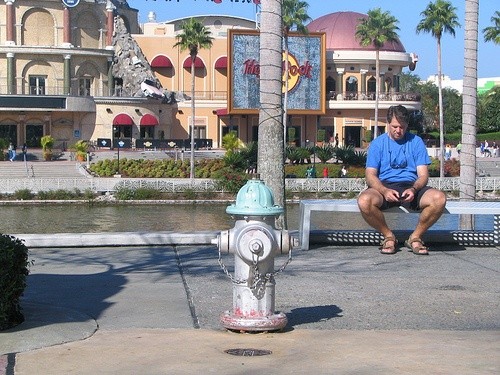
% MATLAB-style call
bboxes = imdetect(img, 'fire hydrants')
[211,173,300,334]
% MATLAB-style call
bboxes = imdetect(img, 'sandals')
[405,235,429,255]
[380,236,399,254]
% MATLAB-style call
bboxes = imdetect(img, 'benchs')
[298,198,499,251]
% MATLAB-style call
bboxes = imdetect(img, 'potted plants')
[41,135,53,160]
[70,139,95,161]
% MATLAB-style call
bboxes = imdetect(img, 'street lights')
[306,139,317,171]
[113,121,119,173]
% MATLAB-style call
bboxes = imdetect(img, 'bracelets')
[412,187,418,196]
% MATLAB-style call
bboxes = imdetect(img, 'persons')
[335,133,339,148]
[7,137,16,162]
[342,165,349,178]
[480,140,485,157]
[456,140,462,153]
[357,105,447,255]
[445,144,451,160]
[484,140,488,154]
